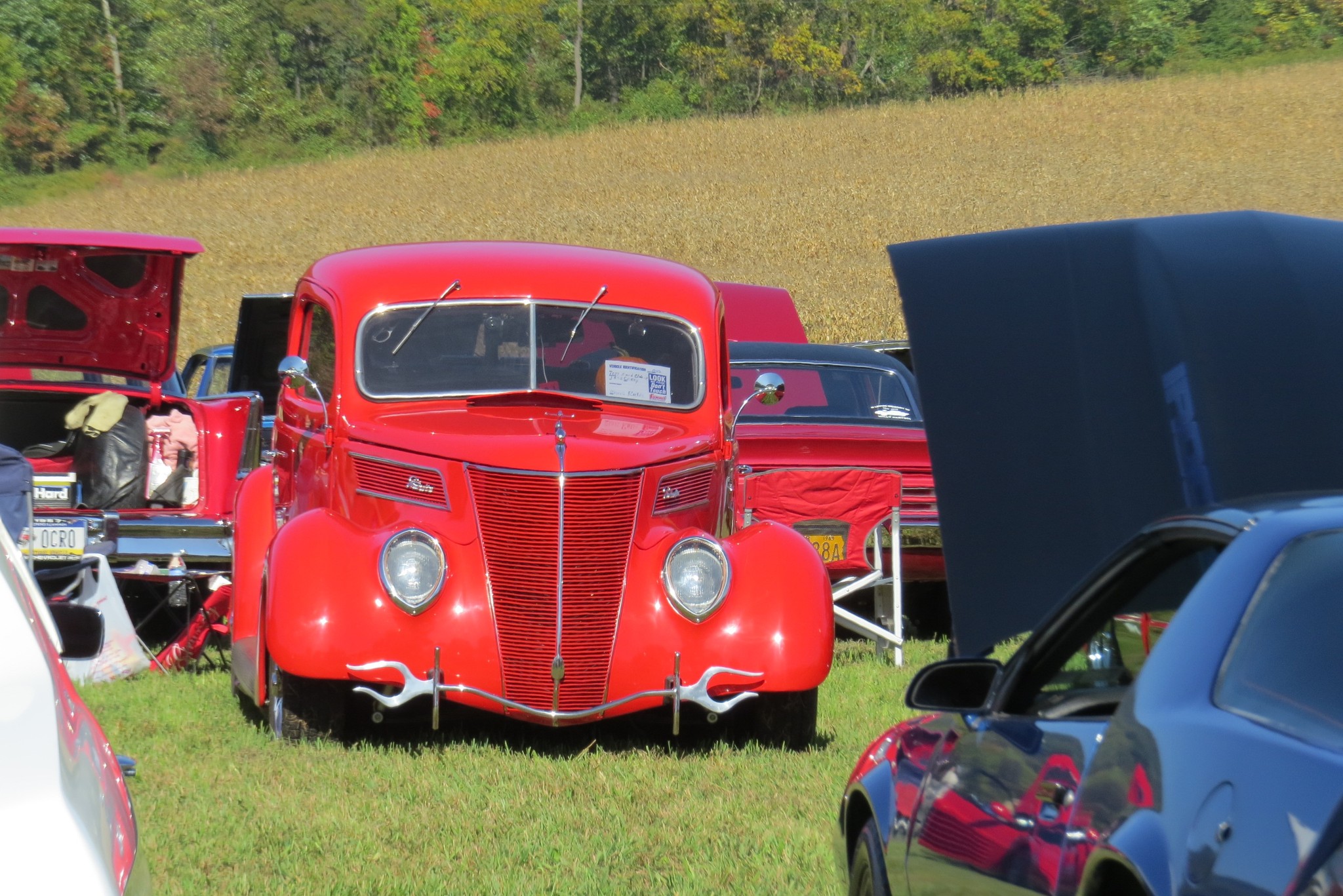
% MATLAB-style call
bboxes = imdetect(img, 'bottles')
[135,560,161,575]
[168,552,187,606]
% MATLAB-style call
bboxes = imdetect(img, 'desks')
[90,568,232,676]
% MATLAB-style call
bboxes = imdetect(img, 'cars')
[225,241,839,753]
[1,228,948,637]
[1,534,141,896]
[839,212,1343,896]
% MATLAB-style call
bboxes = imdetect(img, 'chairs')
[743,467,906,667]
[0,445,99,605]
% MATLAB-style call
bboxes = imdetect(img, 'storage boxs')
[31,473,78,509]
